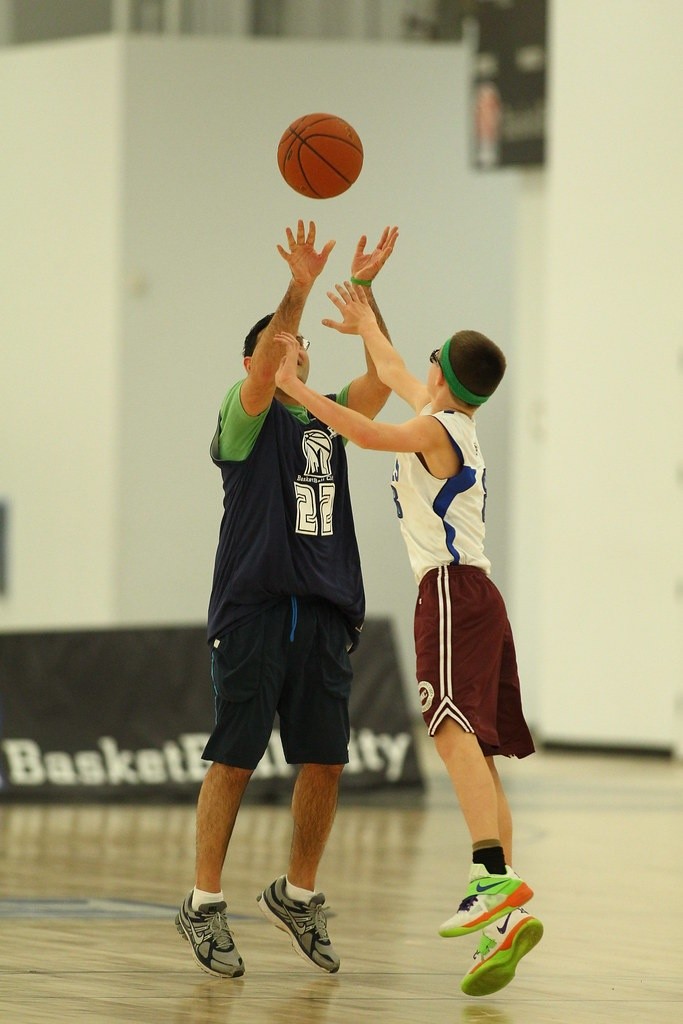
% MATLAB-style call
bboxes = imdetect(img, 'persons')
[272,280,544,998]
[175,218,400,982]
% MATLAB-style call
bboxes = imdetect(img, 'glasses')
[429,349,444,376]
[296,337,310,350]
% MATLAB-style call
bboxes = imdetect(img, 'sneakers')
[460,906,543,996]
[257,875,342,973]
[439,864,537,935]
[175,889,246,978]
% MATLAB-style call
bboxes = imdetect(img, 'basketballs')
[277,113,365,199]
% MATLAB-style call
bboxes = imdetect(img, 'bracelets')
[350,276,374,286]
